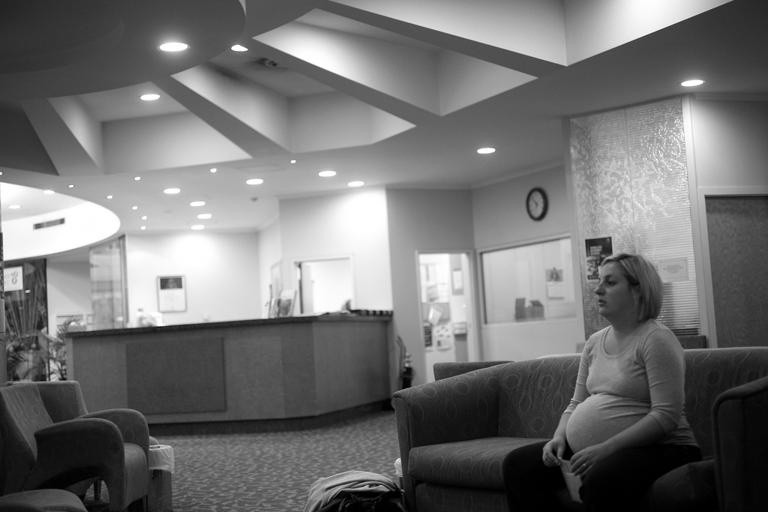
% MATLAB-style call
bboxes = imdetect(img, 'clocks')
[526,188,547,221]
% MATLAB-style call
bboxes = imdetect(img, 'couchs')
[391,345,768,511]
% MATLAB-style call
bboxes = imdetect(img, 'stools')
[0,489,89,512]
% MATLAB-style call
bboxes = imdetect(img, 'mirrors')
[481,238,576,323]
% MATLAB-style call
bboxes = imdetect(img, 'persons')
[503,254,698,512]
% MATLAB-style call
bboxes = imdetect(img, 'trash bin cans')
[148,444,174,511]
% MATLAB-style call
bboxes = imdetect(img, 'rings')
[582,463,587,468]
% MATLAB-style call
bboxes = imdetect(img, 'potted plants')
[6,335,46,381]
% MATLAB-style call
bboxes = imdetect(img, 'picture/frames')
[156,275,187,312]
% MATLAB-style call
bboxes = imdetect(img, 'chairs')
[0,381,149,512]
[433,361,517,381]
[676,335,707,348]
